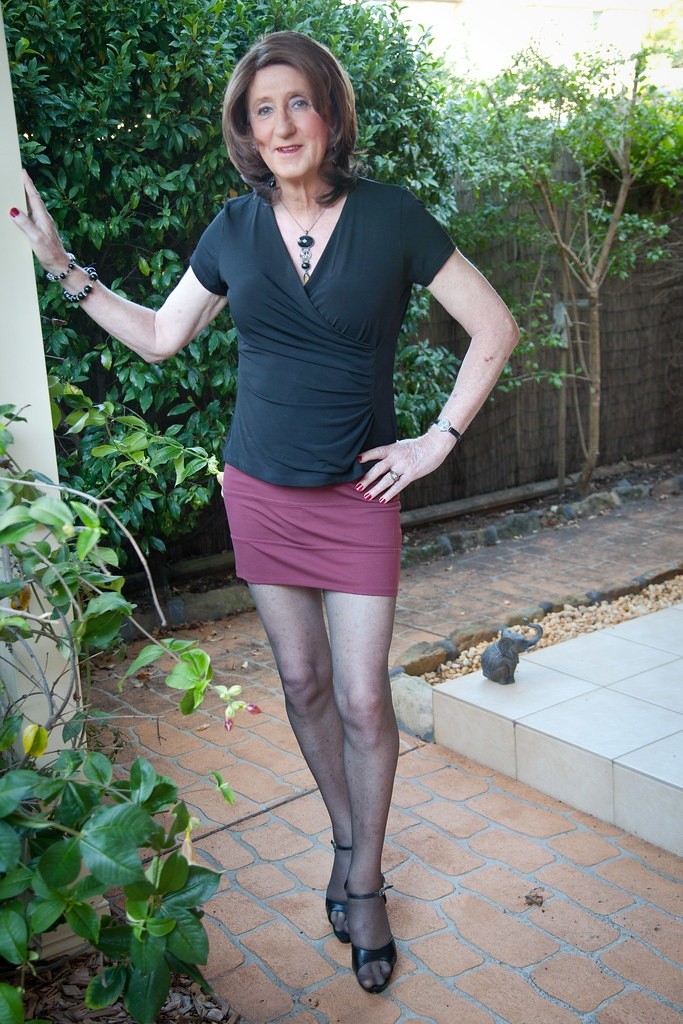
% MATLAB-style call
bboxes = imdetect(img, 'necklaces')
[277,191,328,286]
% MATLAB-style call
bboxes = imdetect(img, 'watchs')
[433,418,462,441]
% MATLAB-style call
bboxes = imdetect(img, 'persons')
[8,29,522,995]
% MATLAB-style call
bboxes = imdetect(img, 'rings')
[389,471,400,482]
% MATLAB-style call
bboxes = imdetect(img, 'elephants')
[480,618,543,686]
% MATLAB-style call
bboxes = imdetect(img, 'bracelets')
[63,266,97,303]
[47,254,76,283]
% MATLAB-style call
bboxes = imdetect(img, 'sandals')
[344,874,398,995]
[326,839,354,944]
